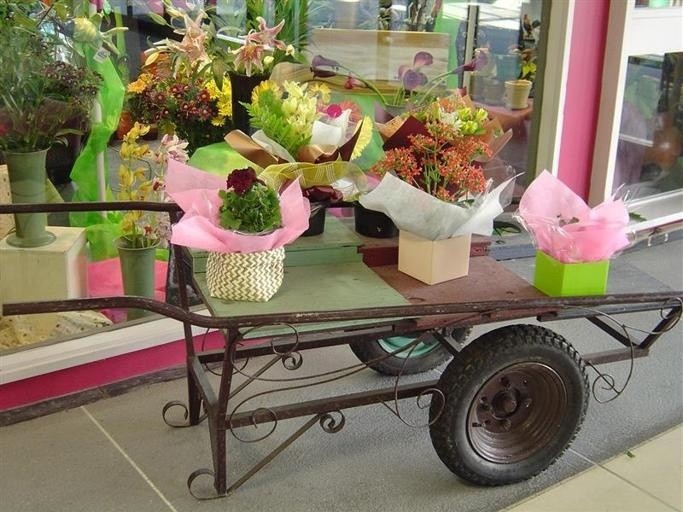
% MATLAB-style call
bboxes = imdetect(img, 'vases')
[349,196,397,239]
[303,188,327,239]
[114,233,161,322]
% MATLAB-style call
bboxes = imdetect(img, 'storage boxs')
[532,249,608,299]
[394,228,473,287]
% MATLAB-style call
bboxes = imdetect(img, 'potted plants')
[504,51,536,112]
[0,1,103,248]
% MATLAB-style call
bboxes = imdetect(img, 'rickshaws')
[1,195,680,500]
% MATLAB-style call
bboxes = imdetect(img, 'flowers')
[384,94,512,235]
[111,127,174,244]
[310,50,492,108]
[215,162,286,235]
[130,15,360,162]
